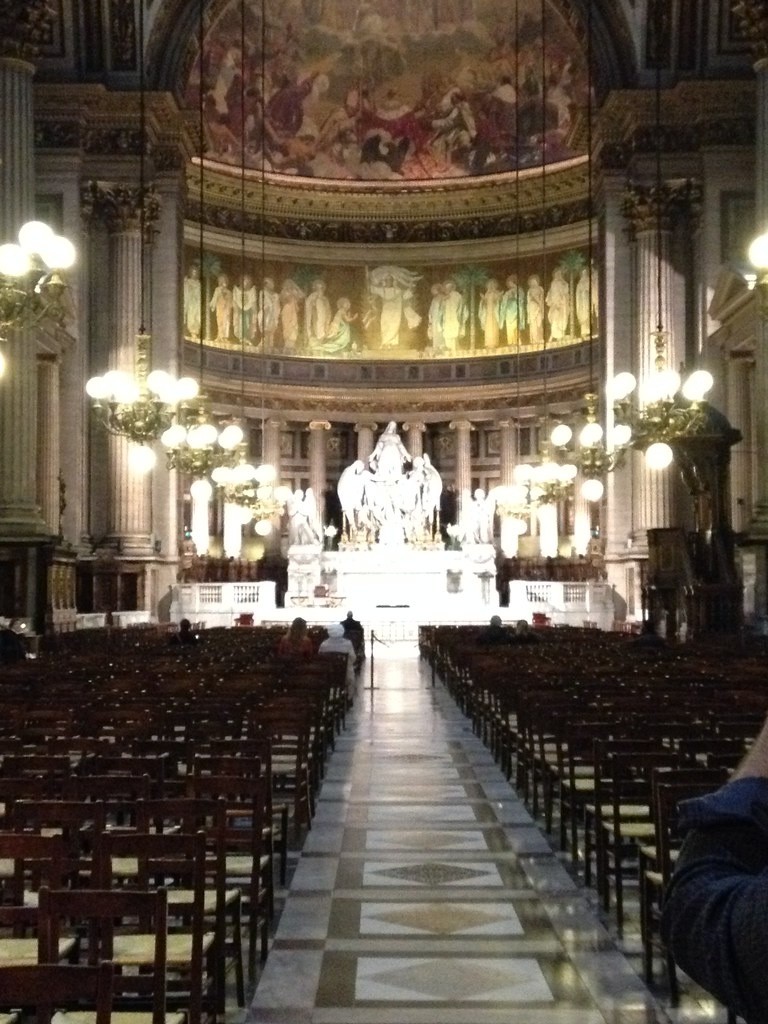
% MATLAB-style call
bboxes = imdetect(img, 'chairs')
[0,628,349,1024]
[420,625,767,1008]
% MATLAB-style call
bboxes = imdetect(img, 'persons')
[657,705,768,1024]
[623,620,667,648]
[201,554,286,583]
[367,420,412,484]
[464,488,491,543]
[296,487,324,544]
[506,620,541,646]
[511,554,588,583]
[0,609,366,709]
[473,615,516,645]
[287,489,319,546]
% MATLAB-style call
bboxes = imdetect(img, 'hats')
[327,624,344,636]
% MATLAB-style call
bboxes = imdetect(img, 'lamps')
[85,0,198,446]
[212,0,288,524]
[0,221,76,341]
[162,0,243,479]
[615,0,714,445]
[551,0,632,478]
[498,0,603,518]
